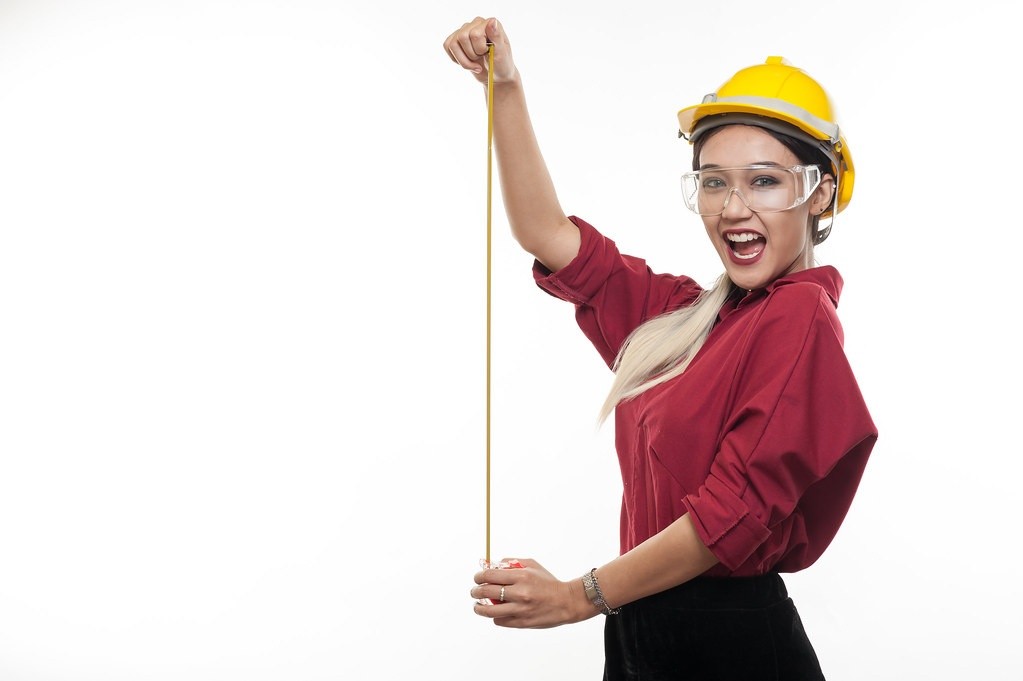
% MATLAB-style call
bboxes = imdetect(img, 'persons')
[442,16,879,681]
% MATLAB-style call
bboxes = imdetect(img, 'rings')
[500,586,504,601]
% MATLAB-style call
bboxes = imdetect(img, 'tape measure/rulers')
[483,36,523,605]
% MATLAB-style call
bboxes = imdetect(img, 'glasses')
[680,164,824,217]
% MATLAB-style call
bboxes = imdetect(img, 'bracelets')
[583,568,622,616]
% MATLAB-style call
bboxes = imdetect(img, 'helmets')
[678,56,856,221]
